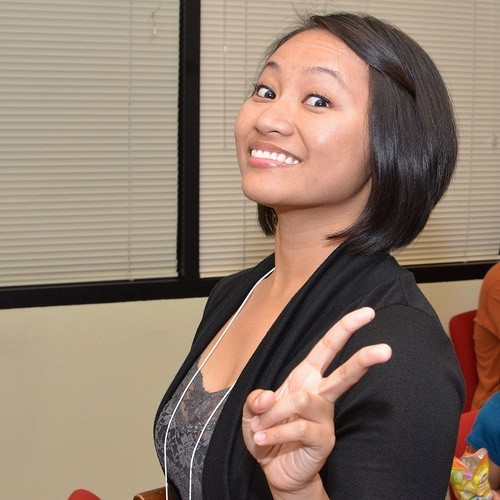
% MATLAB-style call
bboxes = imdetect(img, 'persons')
[151,12,465,500]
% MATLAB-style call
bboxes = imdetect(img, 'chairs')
[447,409,480,500]
[448,308,480,414]
